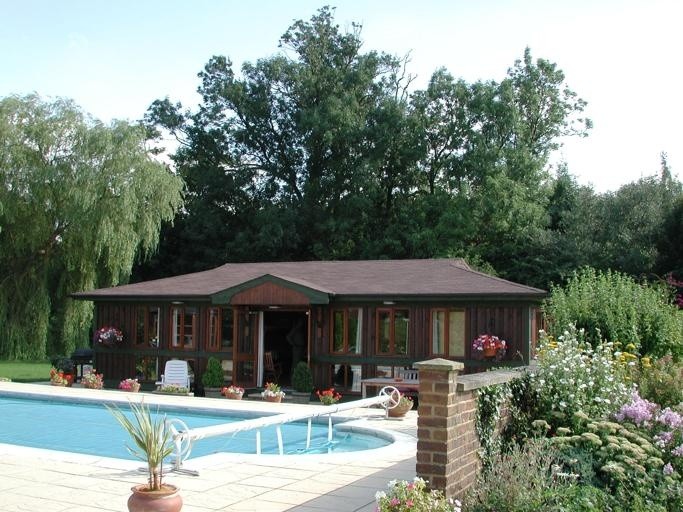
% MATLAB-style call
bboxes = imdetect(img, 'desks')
[356,378,419,400]
[69,351,96,382]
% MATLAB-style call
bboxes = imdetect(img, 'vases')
[378,402,413,417]
[483,348,496,357]
[52,382,64,386]
[263,395,281,403]
[224,393,242,400]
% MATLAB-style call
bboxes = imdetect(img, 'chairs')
[49,357,74,373]
[397,370,419,379]
[154,360,190,391]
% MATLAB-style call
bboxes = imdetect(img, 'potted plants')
[101,400,183,512]
[291,361,315,404]
[152,384,189,397]
[201,356,224,398]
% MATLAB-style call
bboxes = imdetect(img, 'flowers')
[80,368,104,389]
[117,378,141,392]
[316,386,342,404]
[261,381,286,399]
[472,333,508,353]
[219,385,245,400]
[95,326,123,350]
[394,391,413,410]
[48,366,73,386]
